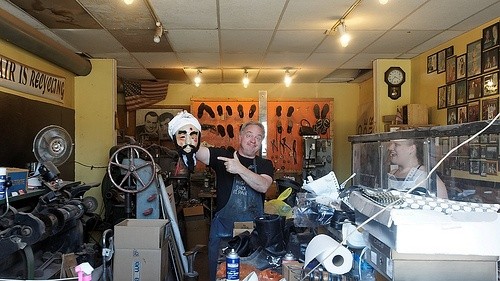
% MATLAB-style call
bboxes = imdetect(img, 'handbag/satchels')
[264,188,293,217]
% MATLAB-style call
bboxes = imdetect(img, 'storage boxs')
[281,259,303,281]
[0,166,28,197]
[112,217,169,280]
[232,221,254,236]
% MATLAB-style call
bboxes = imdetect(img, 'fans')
[25,125,74,187]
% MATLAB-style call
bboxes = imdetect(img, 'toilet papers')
[303,233,353,275]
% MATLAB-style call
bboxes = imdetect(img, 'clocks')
[384,66,407,101]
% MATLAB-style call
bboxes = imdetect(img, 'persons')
[137,111,174,138]
[198,120,274,281]
[388,124,448,198]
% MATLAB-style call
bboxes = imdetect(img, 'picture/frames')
[432,135,500,178]
[426,21,500,125]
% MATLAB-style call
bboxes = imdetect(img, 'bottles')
[354,251,376,281]
[225,248,240,281]
[281,250,297,278]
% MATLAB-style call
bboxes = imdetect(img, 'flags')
[125,80,168,110]
